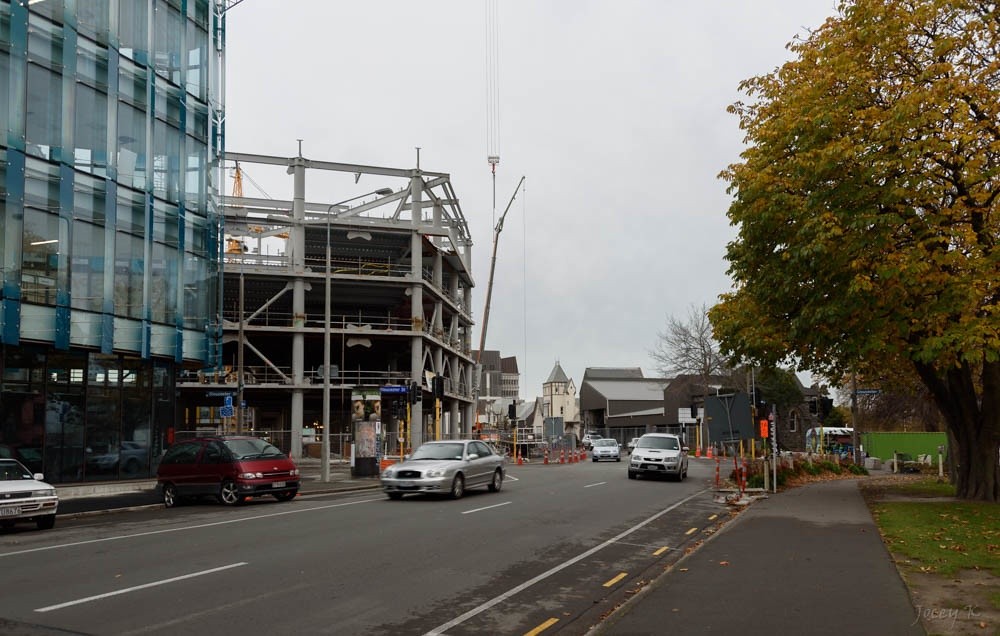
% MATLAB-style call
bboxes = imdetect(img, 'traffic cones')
[707,445,713,457]
[559,447,565,463]
[544,448,549,464]
[517,449,523,464]
[696,445,700,456]
[568,446,588,463]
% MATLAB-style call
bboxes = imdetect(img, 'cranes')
[227,161,293,254]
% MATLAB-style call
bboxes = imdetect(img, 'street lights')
[321,188,393,483]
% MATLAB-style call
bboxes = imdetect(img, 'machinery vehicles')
[475,174,526,445]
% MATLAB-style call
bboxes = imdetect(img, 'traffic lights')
[508,404,515,419]
[808,396,818,417]
[822,398,834,411]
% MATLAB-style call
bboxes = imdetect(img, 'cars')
[91,442,150,475]
[581,434,623,462]
[380,439,507,499]
[1,459,61,531]
[627,432,690,480]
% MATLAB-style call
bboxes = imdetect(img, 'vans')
[806,426,863,454]
[156,435,301,507]
